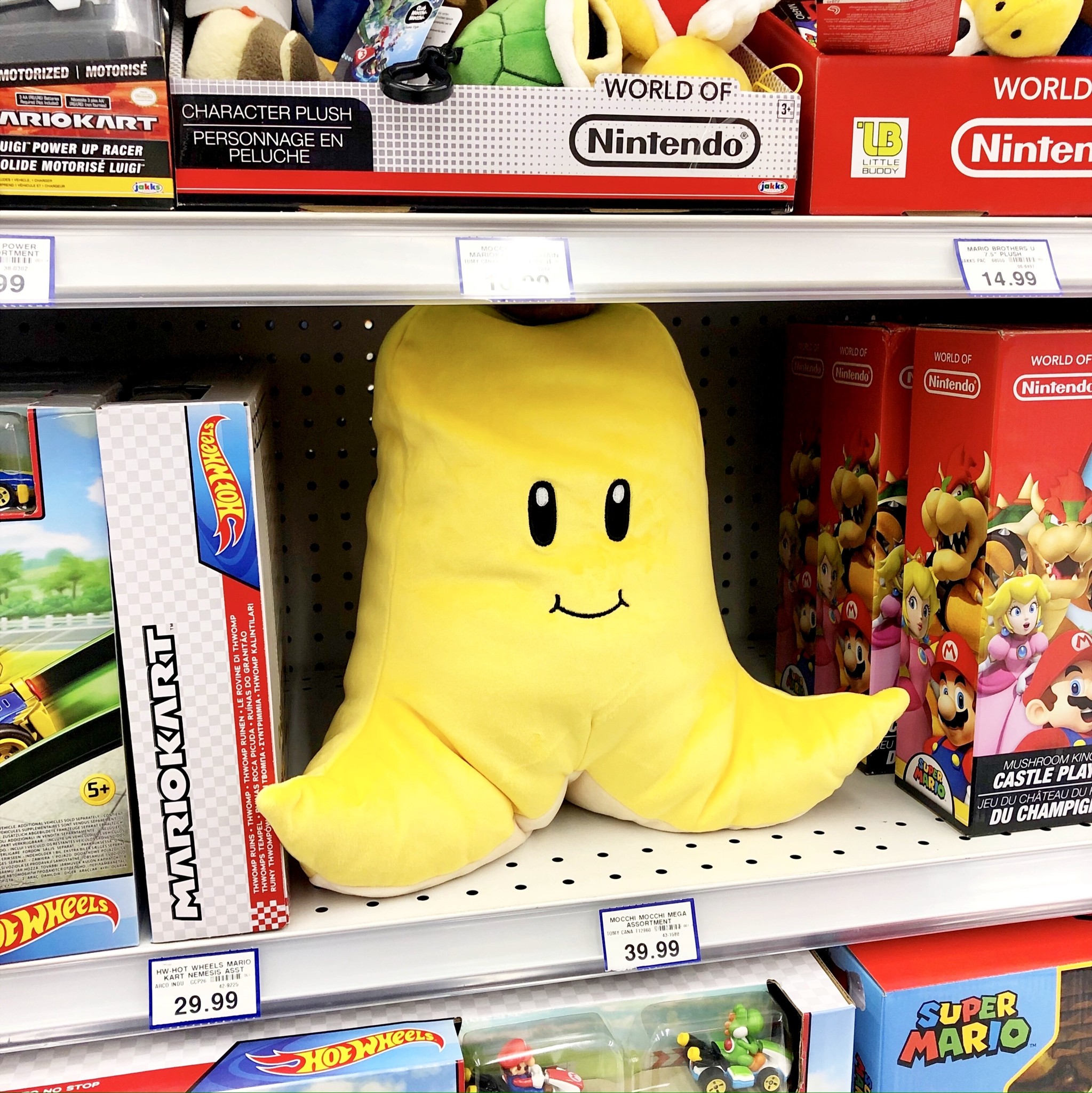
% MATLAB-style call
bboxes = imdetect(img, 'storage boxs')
[0,0,1092,1093]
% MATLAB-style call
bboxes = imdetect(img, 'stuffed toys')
[186,0,371,83]
[950,0,1084,57]
[250,299,909,895]
[440,0,782,91]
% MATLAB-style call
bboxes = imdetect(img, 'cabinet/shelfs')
[0,208,1092,1058]
